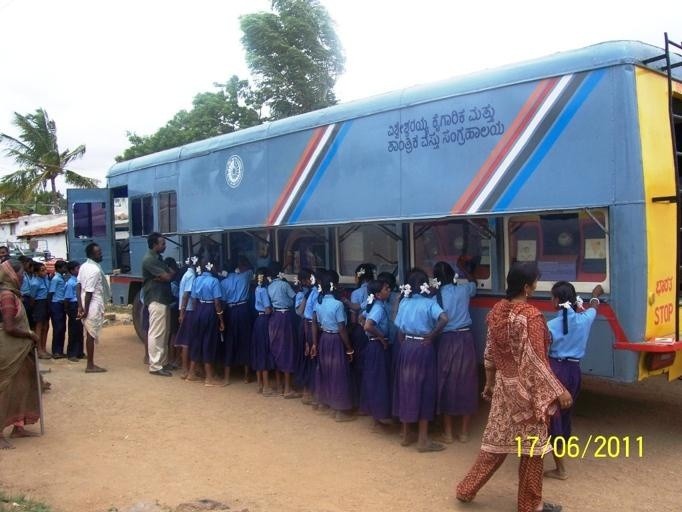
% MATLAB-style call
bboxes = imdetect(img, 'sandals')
[179,369,471,454]
[33,348,108,374]
[543,470,570,480]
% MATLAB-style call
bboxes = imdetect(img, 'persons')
[546,280,604,479]
[453,259,564,511]
[0,241,87,448]
[75,242,111,372]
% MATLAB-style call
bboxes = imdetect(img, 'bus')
[64,40,682,414]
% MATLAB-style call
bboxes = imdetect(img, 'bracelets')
[590,297,600,305]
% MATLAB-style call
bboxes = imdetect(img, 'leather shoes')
[148,366,173,377]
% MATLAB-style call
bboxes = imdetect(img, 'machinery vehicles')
[5,237,62,282]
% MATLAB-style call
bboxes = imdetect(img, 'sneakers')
[524,500,562,512]
